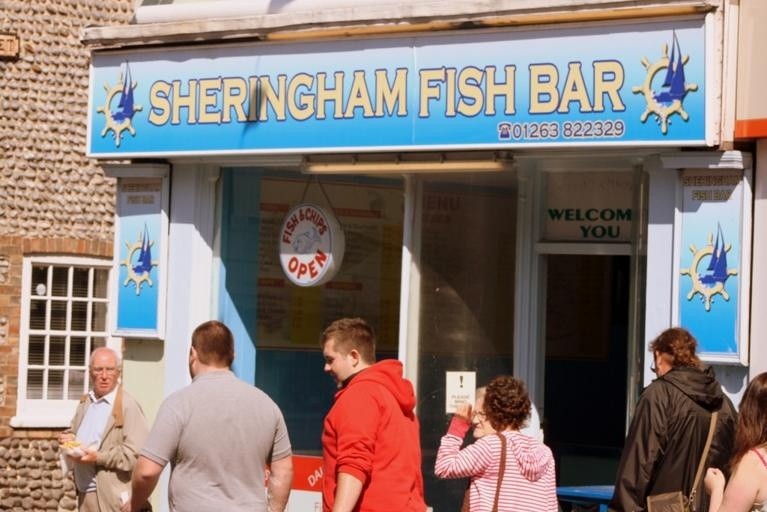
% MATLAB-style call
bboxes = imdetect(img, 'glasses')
[650,361,657,372]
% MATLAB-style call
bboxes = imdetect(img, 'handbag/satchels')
[647,491,690,512]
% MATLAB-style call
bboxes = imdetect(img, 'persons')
[703,372,767,512]
[56,346,153,512]
[457,385,545,512]
[130,319,294,512]
[433,375,559,512]
[606,327,738,512]
[319,317,434,512]
